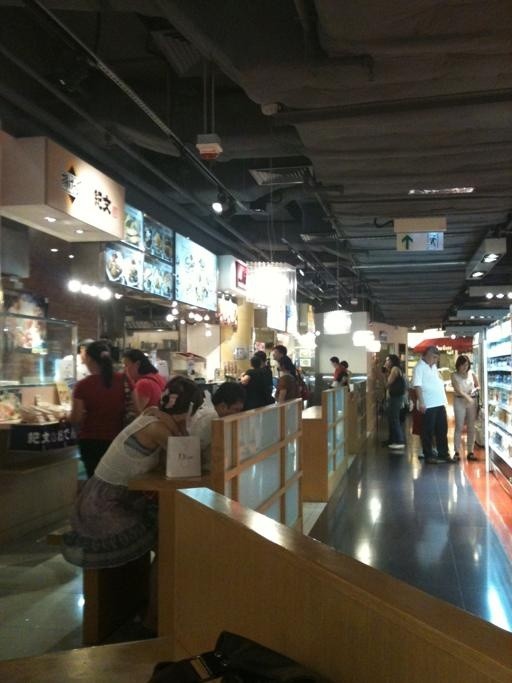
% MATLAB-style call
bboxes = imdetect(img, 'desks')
[5,417,81,536]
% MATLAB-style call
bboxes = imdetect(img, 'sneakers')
[380,438,479,464]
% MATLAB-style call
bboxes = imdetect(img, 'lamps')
[246,157,381,352]
[212,184,227,214]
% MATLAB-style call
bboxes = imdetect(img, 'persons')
[71,340,126,477]
[254,349,275,405]
[239,355,265,411]
[329,355,354,393]
[384,452,485,594]
[123,347,167,412]
[69,372,203,644]
[273,356,300,404]
[450,353,481,461]
[190,381,247,449]
[411,344,453,463]
[378,353,415,450]
[272,345,297,378]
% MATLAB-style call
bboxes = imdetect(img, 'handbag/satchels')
[165,434,202,479]
[388,374,406,398]
[295,374,308,397]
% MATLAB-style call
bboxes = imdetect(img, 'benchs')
[0,488,512,683]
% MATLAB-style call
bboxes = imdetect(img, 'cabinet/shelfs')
[301,387,351,503]
[482,317,512,500]
[352,377,391,451]
[132,398,303,538]
